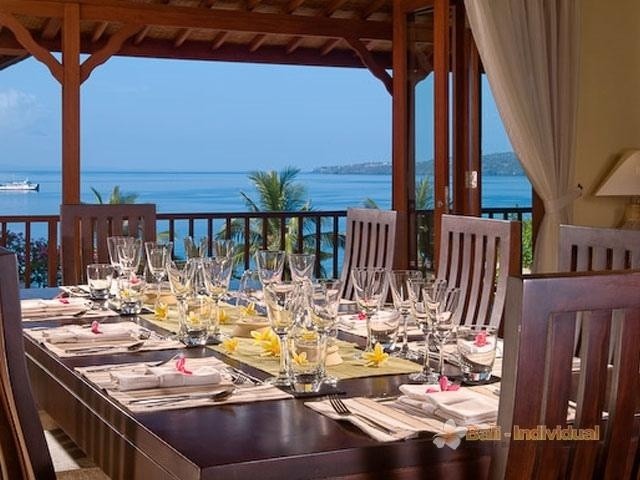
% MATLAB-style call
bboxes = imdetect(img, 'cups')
[115,277,147,316]
[87,265,116,298]
[106,236,172,305]
[454,325,498,384]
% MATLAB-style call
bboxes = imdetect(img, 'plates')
[400,380,500,419]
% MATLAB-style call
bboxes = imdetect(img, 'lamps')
[594,150,640,230]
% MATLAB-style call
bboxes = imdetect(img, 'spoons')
[133,326,153,341]
[61,341,150,353]
[22,309,90,320]
[128,389,234,407]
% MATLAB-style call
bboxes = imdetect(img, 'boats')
[1,179,39,192]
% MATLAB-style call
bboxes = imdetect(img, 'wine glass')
[199,258,233,344]
[303,280,342,383]
[424,289,462,387]
[350,266,391,363]
[387,270,425,361]
[406,277,448,383]
[165,260,195,341]
[263,283,302,387]
[180,297,213,345]
[289,254,317,283]
[255,251,287,289]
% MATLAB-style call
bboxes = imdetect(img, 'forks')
[81,352,182,372]
[330,394,395,438]
[217,361,251,387]
